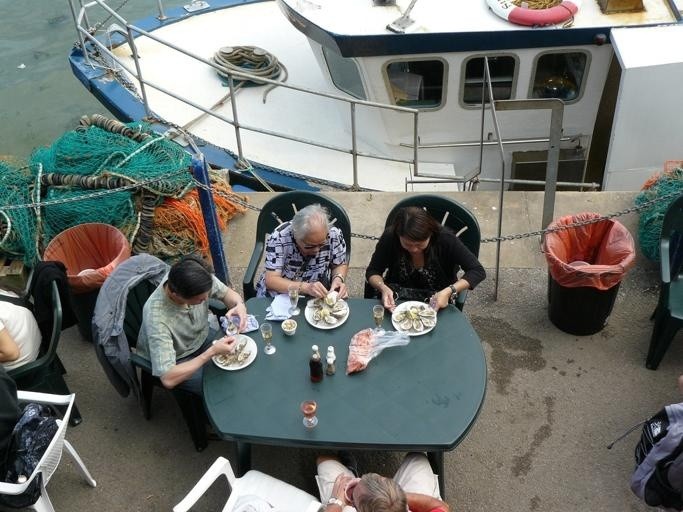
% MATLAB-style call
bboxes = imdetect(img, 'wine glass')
[372,305,385,334]
[260,323,277,354]
[301,400,318,427]
[288,283,301,316]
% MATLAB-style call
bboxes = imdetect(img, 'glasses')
[301,236,328,249]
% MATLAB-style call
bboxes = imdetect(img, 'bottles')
[309,345,324,382]
[326,346,337,375]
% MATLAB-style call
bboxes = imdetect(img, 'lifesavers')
[486,0,583,27]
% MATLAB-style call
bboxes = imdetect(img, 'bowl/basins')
[281,319,297,336]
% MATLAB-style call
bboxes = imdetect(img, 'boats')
[60,1,682,191]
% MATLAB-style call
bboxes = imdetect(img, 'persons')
[256,203,350,300]
[315,452,450,512]
[136,257,248,443]
[0,299,44,386]
[365,206,487,312]
[0,364,18,482]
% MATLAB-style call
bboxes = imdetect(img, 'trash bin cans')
[44,222,130,343]
[544,212,636,336]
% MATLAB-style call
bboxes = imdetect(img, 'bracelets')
[298,281,305,295]
[331,272,344,282]
[328,497,343,507]
[449,284,458,299]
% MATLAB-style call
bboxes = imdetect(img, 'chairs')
[0,267,96,512]
[641,194,683,370]
[243,192,480,313]
[93,254,229,453]
[173,458,323,511]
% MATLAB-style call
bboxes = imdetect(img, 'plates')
[390,300,436,337]
[305,296,349,330]
[211,334,258,371]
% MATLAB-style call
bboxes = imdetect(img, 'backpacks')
[608,403,683,512]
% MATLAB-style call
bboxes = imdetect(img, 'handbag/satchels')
[1,400,58,509]
